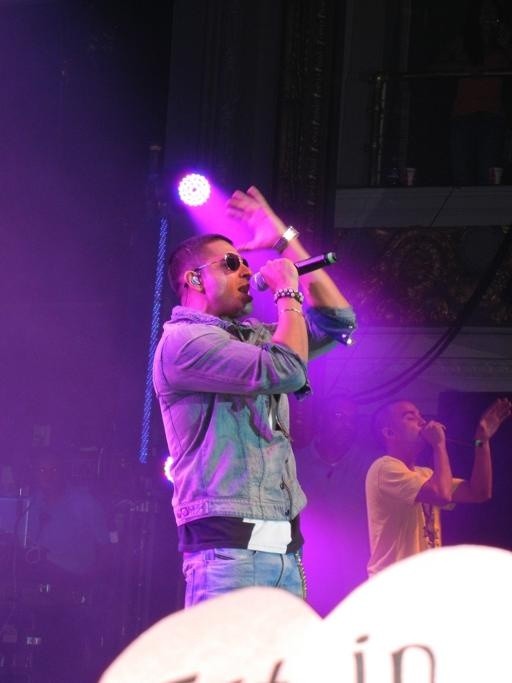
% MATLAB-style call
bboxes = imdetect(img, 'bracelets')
[272,224,300,253]
[274,290,304,304]
[279,306,306,318]
[471,439,483,447]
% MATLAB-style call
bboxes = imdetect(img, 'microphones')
[444,430,484,447]
[254,251,337,291]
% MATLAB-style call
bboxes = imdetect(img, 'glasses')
[185,252,248,289]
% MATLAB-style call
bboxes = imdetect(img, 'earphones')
[191,276,201,285]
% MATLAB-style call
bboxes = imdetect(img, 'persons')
[152,186,356,610]
[365,397,512,579]
